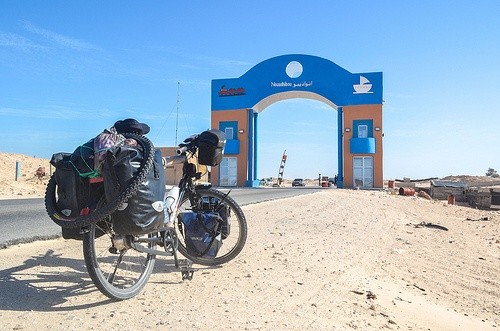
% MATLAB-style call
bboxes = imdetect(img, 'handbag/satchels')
[177,213,222,260]
[195,128,226,166]
[48,125,180,240]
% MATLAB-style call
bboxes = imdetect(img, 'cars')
[259,178,273,186]
[292,178,306,187]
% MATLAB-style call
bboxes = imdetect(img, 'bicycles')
[82,117,247,299]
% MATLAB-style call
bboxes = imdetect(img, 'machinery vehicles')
[273,149,287,187]
[322,177,332,187]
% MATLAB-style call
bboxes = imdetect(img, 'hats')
[116,118,151,134]
